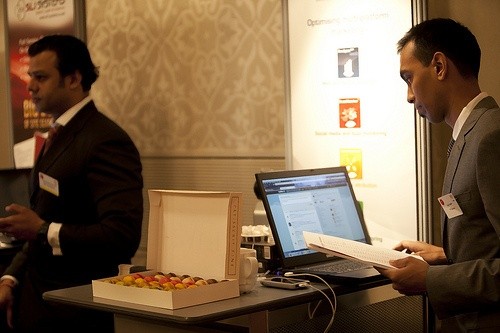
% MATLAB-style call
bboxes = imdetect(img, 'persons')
[372,17,500,333]
[0,32,145,333]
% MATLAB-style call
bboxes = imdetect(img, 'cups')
[238,248,258,293]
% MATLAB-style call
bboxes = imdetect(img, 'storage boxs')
[91,189,242,310]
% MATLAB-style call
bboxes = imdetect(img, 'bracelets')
[1,283,13,287]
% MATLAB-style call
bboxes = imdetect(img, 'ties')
[43,124,62,156]
[445,139,455,159]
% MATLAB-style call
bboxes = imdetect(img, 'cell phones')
[260,276,310,289]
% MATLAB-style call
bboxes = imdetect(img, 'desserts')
[102,271,230,291]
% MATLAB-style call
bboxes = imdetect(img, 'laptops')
[256,166,385,283]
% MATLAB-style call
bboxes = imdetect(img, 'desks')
[43,276,435,333]
